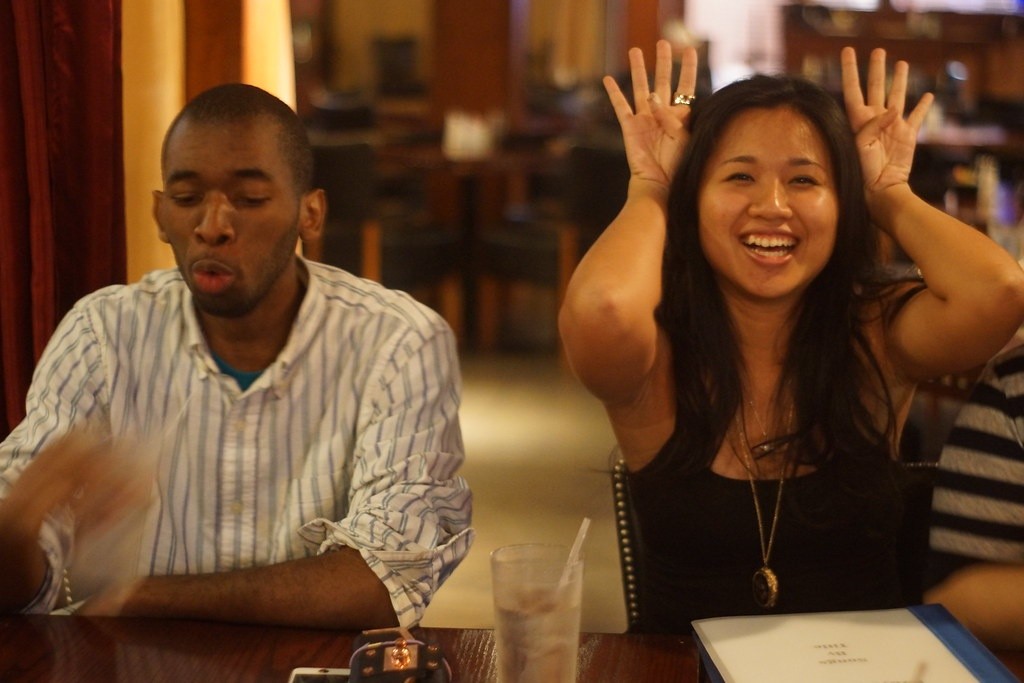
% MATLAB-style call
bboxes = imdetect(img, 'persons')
[1,83,472,631]
[558,33,1024,651]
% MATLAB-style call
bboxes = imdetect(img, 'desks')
[374,133,572,353]
[0,606,1024,683]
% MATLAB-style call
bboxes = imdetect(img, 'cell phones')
[288,667,350,683]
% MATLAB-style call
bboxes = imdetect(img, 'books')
[686,604,1024,683]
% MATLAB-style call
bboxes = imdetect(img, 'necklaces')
[748,390,784,452]
[728,385,797,609]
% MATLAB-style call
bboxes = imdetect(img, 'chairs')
[294,86,630,353]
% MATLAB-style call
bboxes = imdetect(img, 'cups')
[64,471,160,617]
[490,542,583,683]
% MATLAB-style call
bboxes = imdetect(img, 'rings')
[673,92,695,106]
